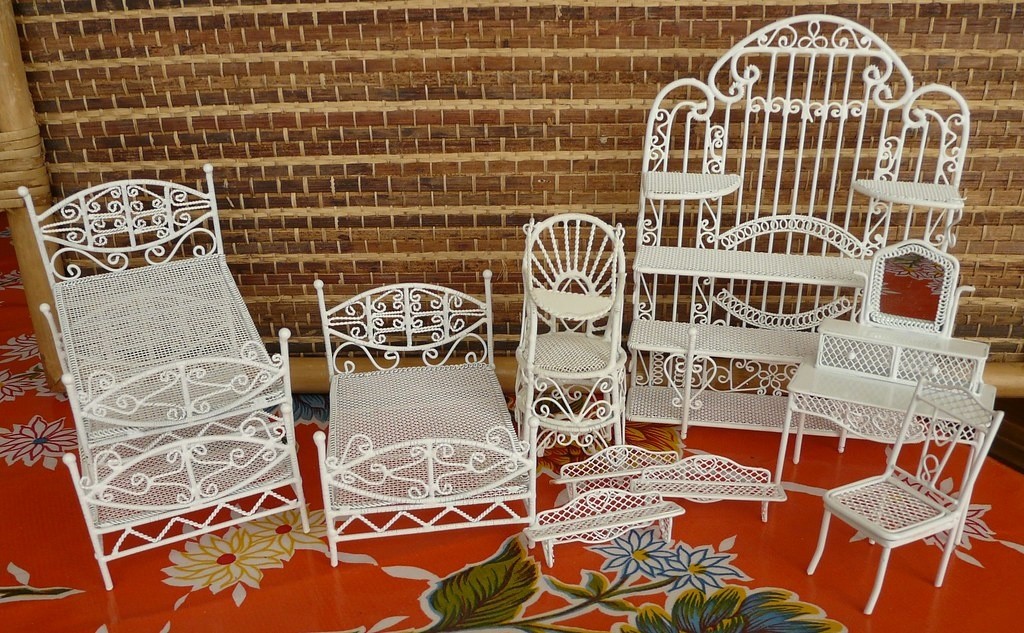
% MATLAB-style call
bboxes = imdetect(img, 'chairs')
[807,373,1007,616]
[512,213,630,464]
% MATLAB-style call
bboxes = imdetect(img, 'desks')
[774,319,990,496]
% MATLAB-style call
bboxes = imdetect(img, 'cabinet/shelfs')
[624,12,990,444]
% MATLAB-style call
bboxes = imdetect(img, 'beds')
[18,162,311,592]
[313,269,541,567]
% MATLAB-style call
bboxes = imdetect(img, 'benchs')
[525,444,789,566]
